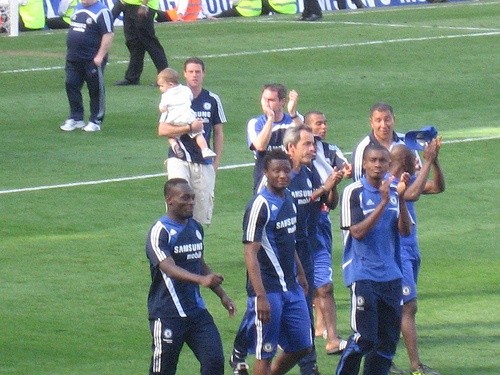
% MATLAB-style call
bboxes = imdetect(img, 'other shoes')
[172,142,184,158]
[296,13,322,21]
[202,149,216,158]
[113,79,139,86]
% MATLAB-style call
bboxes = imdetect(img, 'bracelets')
[141,5,147,8]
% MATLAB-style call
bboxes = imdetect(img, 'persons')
[60,0,114,132]
[46,0,78,29]
[115,0,168,87]
[212,0,262,18]
[145,178,235,375]
[228,83,352,375]
[296,0,323,21]
[156,56,227,228]
[155,0,217,22]
[18,0,45,31]
[261,0,297,15]
[335,103,446,375]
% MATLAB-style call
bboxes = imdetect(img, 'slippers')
[326,340,347,354]
[315,329,342,339]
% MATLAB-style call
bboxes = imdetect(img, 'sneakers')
[60,119,85,131]
[82,122,101,131]
[410,363,438,375]
[228,355,249,375]
[388,362,406,374]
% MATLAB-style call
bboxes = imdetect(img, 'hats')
[405,126,437,151]
